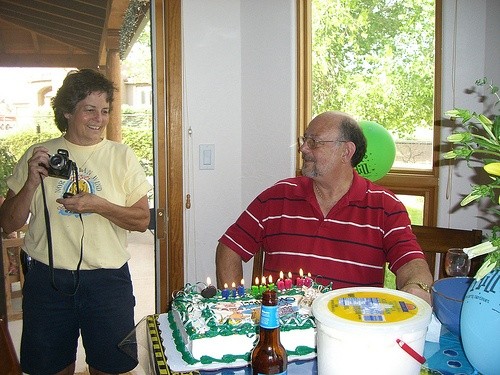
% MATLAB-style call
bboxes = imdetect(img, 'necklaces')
[314,185,345,203]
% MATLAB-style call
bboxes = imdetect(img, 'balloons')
[459,269,500,375]
[351,119,395,183]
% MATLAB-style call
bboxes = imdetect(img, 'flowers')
[443,76,500,281]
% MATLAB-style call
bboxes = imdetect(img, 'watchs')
[399,281,431,293]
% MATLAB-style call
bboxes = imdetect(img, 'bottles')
[251,289,288,375]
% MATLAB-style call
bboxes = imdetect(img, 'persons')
[0,67,150,375]
[213,111,434,320]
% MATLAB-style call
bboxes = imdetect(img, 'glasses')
[298,136,349,150]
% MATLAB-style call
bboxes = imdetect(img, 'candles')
[200,269,315,300]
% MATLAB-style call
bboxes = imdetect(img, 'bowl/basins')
[431,277,475,337]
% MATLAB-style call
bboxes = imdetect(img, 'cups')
[445,248,471,278]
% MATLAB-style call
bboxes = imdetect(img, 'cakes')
[168,281,332,365]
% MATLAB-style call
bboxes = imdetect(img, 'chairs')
[411,224,483,284]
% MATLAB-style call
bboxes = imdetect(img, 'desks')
[145,313,481,375]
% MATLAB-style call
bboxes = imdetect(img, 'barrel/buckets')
[311,286,433,375]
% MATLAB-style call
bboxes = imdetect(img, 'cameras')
[39,149,75,180]
